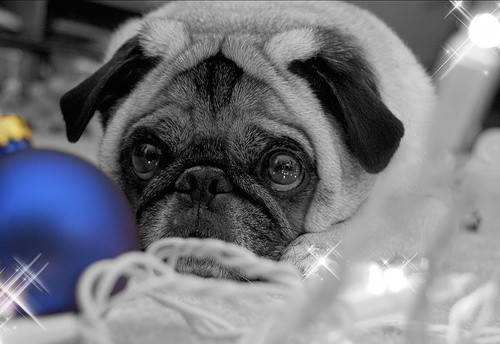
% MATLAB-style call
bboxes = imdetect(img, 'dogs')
[59,0,441,283]
